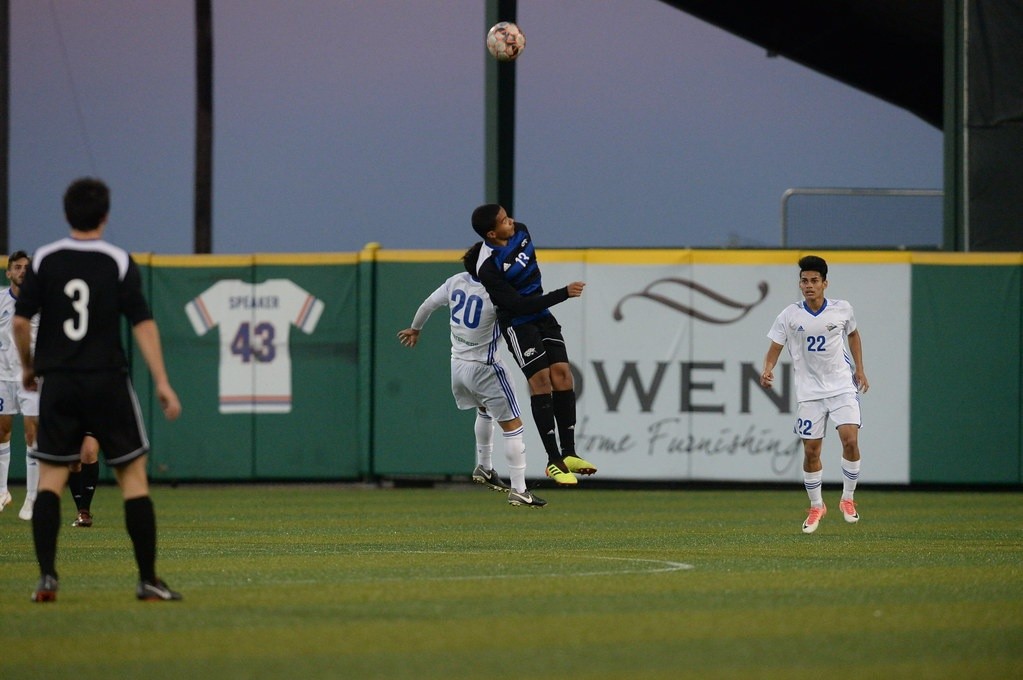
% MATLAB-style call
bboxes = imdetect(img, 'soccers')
[487,21,526,60]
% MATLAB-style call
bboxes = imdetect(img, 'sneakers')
[138,578,182,602]
[31,575,57,601]
[839,498,859,523]
[472,464,510,493]
[545,462,578,486]
[562,454,598,476]
[507,488,548,509]
[0,491,12,512]
[18,496,36,520]
[802,502,827,534]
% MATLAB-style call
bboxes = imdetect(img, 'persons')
[11,177,183,601]
[761,256,869,533]
[0,250,100,526]
[470,204,597,487]
[397,242,548,509]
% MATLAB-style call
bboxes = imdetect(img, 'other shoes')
[78,509,92,527]
[71,519,78,527]
